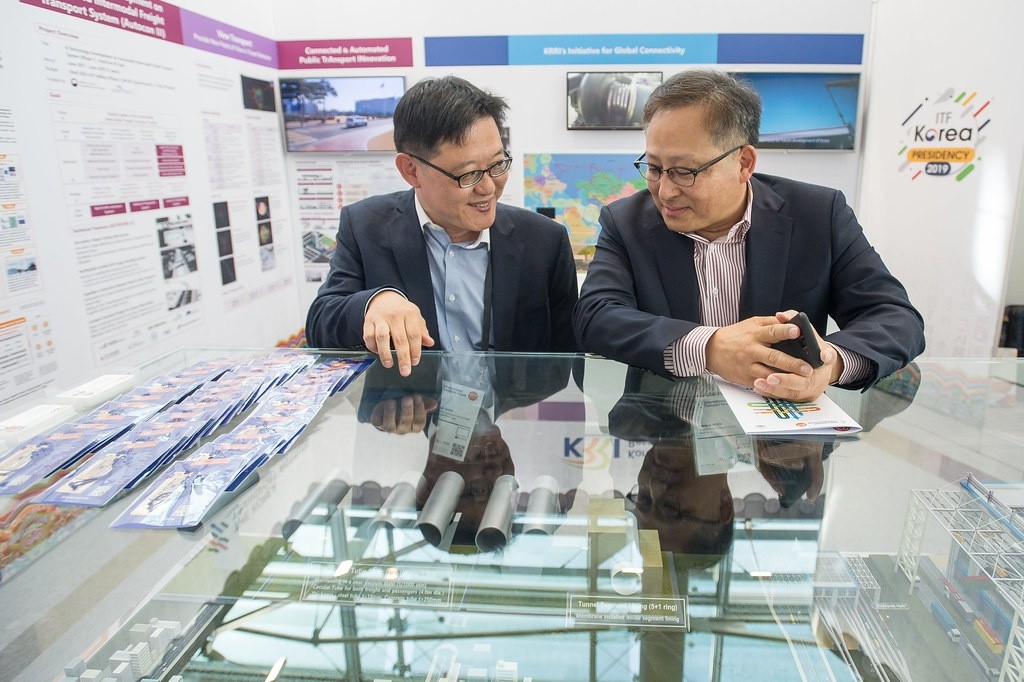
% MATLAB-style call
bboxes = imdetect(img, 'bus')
[345,115,367,128]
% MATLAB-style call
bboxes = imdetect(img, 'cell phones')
[760,312,821,374]
[769,454,815,509]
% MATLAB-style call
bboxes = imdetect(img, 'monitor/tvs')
[713,63,869,158]
[279,75,407,153]
[565,72,664,131]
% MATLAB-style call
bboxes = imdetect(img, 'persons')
[305,76,579,376]
[609,362,922,572]
[358,356,571,556]
[574,71,926,402]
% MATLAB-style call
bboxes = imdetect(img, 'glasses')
[633,145,745,188]
[626,484,720,524]
[409,149,513,188]
[461,477,494,499]
